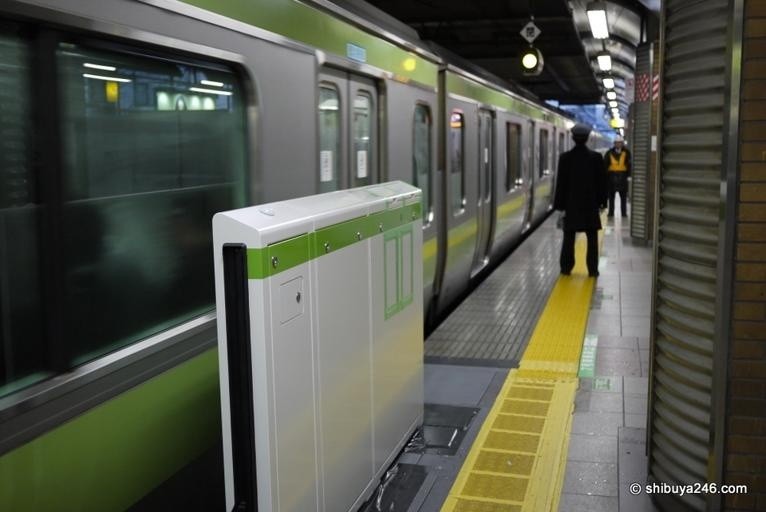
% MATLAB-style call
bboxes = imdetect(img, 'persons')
[49,203,164,358]
[602,133,634,218]
[551,122,607,277]
[158,190,232,320]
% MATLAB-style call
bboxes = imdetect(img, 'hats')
[571,122,592,135]
[612,135,623,143]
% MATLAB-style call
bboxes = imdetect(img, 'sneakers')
[608,211,627,217]
[559,268,599,277]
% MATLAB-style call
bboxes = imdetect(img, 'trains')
[0,0,614,510]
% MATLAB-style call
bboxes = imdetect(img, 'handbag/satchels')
[557,214,566,230]
